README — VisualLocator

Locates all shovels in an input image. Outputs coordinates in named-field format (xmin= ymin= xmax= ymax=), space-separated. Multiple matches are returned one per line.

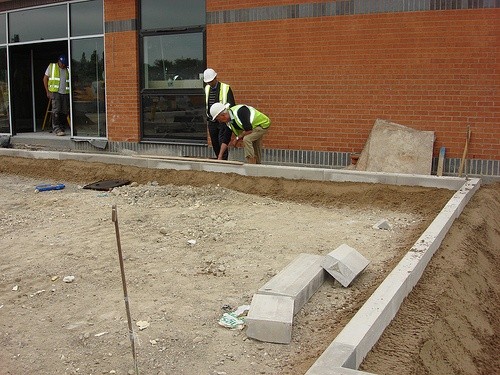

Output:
xmin=123 ymin=149 xmax=244 ymax=164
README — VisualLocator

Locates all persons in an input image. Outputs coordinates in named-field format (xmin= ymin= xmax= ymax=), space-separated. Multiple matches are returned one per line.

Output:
xmin=210 ymin=103 xmax=272 ymax=164
xmin=43 ymin=55 xmax=69 ymax=136
xmin=204 ymin=68 xmax=235 ymax=160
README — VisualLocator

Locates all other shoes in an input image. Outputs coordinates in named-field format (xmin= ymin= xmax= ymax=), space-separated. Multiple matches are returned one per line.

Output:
xmin=54 ymin=130 xmax=66 ymax=136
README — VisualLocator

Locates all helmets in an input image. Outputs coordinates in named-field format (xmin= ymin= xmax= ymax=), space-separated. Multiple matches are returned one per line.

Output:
xmin=204 ymin=69 xmax=217 ymax=82
xmin=58 ymin=55 xmax=68 ymax=65
xmin=209 ymin=103 xmax=230 ymax=120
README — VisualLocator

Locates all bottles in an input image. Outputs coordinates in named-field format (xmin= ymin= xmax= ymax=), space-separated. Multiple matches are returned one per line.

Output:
xmin=168 ymin=78 xmax=173 ymax=87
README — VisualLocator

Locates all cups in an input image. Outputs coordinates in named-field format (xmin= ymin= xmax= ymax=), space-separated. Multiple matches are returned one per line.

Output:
xmin=199 ymin=74 xmax=204 ymax=79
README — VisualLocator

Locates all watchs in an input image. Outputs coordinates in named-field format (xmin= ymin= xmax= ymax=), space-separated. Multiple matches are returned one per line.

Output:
xmin=236 ymin=137 xmax=239 ymax=140
